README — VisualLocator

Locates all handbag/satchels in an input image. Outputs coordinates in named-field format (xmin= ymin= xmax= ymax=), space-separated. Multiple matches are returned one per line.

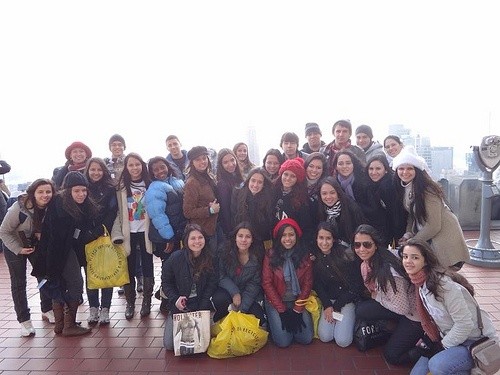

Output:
xmin=354 ymin=319 xmax=392 ymax=352
xmin=206 ymin=310 xmax=270 ymax=359
xmin=85 ymin=224 xmax=130 ymax=290
xmin=171 ymin=304 xmax=211 ymax=357
xmin=295 ymin=295 xmax=323 ymax=340
xmin=469 ymin=336 xmax=500 ymax=375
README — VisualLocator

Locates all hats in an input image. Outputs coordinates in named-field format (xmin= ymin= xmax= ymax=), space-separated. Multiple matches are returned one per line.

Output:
xmin=273 ymin=218 xmax=302 ymax=239
xmin=304 ymin=123 xmax=323 ymax=138
xmin=355 ymin=125 xmax=373 ymax=139
xmin=65 ymin=142 xmax=92 ymax=161
xmin=188 ymin=146 xmax=210 ymax=160
xmin=332 ymin=119 xmax=353 ymax=136
xmin=391 ymin=145 xmax=426 ymax=172
xmin=108 ymin=134 xmax=126 ymax=152
xmin=279 ymin=157 xmax=306 ymax=184
xmin=63 ymin=171 xmax=88 ymax=188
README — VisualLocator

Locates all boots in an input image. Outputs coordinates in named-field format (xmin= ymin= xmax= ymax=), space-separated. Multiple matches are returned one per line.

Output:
xmin=124 ymin=278 xmax=136 ymax=319
xmin=52 ymin=295 xmax=93 ymax=336
xmin=140 ymin=276 xmax=154 ymax=316
xmin=136 ymin=273 xmax=144 ymax=292
xmin=118 ymin=285 xmax=124 ymax=294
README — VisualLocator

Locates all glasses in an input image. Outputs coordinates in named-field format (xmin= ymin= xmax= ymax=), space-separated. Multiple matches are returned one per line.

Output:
xmin=352 ymin=241 xmax=375 ymax=249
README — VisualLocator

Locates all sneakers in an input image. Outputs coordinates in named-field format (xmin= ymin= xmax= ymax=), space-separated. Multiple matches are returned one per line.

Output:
xmin=41 ymin=309 xmax=56 ymax=324
xmin=88 ymin=303 xmax=110 ymax=325
xmin=19 ymin=319 xmax=36 ymax=337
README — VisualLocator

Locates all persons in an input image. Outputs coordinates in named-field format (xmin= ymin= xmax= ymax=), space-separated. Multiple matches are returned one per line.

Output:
xmin=0 ymin=120 xmax=471 ymax=365
xmin=399 ymin=238 xmax=497 ymax=375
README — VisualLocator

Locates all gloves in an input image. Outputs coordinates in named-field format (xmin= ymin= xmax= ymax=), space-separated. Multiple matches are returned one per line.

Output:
xmin=93 ymin=223 xmax=112 ymax=236
xmin=280 ymin=309 xmax=306 ymax=334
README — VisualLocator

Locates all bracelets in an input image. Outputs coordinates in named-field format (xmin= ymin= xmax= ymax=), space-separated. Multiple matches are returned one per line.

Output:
xmin=210 ymin=207 xmax=214 ymax=214
